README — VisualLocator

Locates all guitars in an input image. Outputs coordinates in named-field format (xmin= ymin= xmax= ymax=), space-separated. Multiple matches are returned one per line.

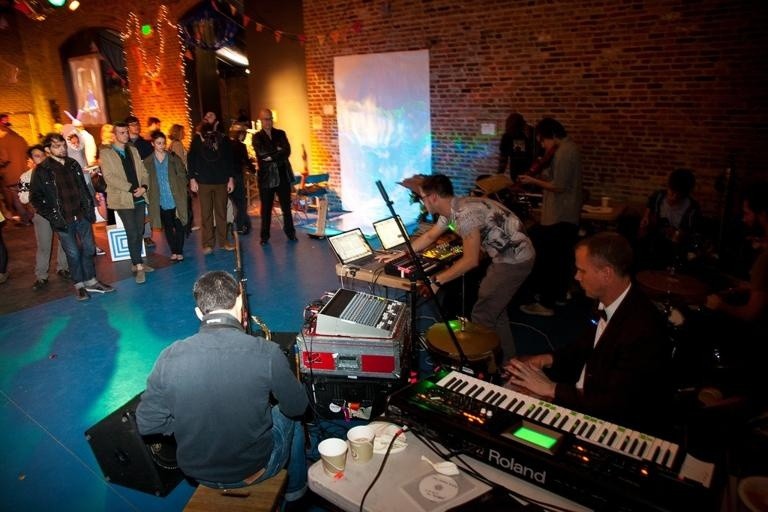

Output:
xmin=639 ymin=211 xmax=691 ymax=245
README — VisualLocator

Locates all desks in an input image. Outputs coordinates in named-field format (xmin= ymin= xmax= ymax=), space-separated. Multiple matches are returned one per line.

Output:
xmin=531 ymin=200 xmax=625 ymax=223
xmin=637 ymin=266 xmax=702 ymax=315
xmin=306 ymin=419 xmax=493 ymax=512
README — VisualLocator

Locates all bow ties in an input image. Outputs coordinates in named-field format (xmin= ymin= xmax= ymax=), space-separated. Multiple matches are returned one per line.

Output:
xmin=594 ymin=308 xmax=608 ymax=322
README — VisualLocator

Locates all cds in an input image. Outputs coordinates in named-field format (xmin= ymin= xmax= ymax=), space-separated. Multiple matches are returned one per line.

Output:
xmin=418 ymin=473 xmax=460 ymax=503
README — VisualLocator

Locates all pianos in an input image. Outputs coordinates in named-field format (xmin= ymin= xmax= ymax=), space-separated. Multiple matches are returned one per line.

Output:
xmin=385 ymin=368 xmax=727 ymax=512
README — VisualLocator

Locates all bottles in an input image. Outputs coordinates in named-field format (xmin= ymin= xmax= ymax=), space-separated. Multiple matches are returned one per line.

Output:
xmin=255 ymin=120 xmax=261 ymax=129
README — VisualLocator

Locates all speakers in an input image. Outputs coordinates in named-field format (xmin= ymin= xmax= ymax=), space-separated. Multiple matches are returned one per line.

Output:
xmin=85 ymin=391 xmax=187 ymax=499
xmin=252 ymin=331 xmax=299 ymax=406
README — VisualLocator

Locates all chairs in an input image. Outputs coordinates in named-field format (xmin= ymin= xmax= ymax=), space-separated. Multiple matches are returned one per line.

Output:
xmin=258 ymin=178 xmax=309 ymax=228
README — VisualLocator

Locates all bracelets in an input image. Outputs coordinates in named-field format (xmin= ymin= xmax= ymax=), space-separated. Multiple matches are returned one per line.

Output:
xmin=431 ymin=276 xmax=442 ymax=288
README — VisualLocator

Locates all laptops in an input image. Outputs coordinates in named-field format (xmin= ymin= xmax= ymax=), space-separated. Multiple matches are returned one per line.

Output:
xmin=373 ymin=215 xmax=436 ymax=257
xmin=326 ymin=227 xmax=404 ymax=274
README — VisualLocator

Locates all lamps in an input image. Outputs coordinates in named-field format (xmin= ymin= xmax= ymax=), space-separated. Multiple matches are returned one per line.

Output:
xmin=206 ymin=45 xmax=256 ymax=78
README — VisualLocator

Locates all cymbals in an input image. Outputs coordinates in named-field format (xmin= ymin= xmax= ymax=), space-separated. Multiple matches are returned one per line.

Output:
xmin=636 ymin=270 xmax=705 ymax=296
xmin=427 ymin=320 xmax=501 ymax=361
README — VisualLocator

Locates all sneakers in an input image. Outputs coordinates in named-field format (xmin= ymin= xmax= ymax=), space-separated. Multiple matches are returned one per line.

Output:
xmin=0 ymin=246 xmax=115 ymax=301
xmin=131 ymin=224 xmax=298 ymax=284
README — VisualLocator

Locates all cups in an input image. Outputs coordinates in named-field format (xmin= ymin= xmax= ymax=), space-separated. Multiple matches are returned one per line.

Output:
xmin=600 ymin=196 xmax=611 ymax=206
xmin=316 ymin=437 xmax=349 ymax=478
xmin=345 ymin=424 xmax=379 ymax=463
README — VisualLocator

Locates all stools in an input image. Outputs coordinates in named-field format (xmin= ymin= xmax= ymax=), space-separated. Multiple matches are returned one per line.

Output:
xmin=182 ymin=468 xmax=288 ymax=511
xmin=426 ymin=320 xmax=505 ymax=388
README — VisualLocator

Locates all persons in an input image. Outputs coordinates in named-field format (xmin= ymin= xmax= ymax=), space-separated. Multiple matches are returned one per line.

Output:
xmin=704 ymin=184 xmax=767 ymax=322
xmin=640 ymin=169 xmax=707 ymax=246
xmin=373 ymin=174 xmax=536 ymax=367
xmin=500 ymin=232 xmax=663 ymax=440
xmin=135 ymin=269 xmax=309 ymax=507
xmin=84 ymin=79 xmax=102 ymax=119
xmin=495 ymin=111 xmax=585 ymax=316
xmin=17 ymin=108 xmax=298 ymax=302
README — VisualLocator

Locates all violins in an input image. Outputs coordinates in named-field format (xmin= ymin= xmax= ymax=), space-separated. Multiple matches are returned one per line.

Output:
xmin=504 ymin=143 xmax=558 ymax=197
